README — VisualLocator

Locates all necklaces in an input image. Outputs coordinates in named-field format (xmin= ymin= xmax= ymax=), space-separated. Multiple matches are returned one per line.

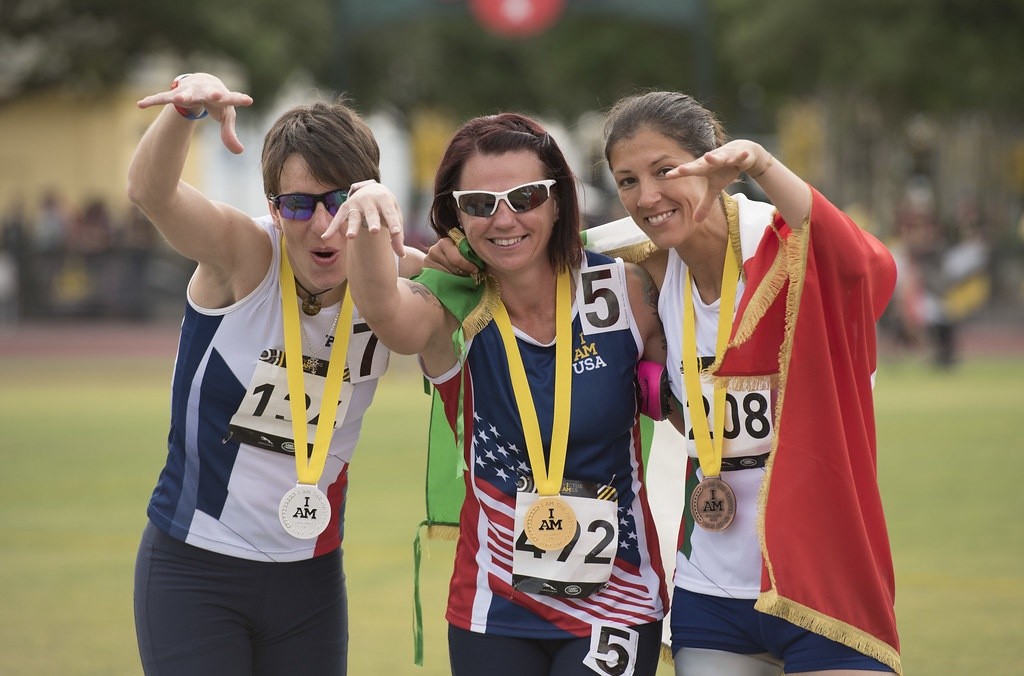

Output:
xmin=292 ymin=273 xmax=346 ymax=369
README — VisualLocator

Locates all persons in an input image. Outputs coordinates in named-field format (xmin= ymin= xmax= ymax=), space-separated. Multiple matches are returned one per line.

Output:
xmin=320 ymin=108 xmax=674 ymax=676
xmin=605 ymin=88 xmax=901 ymax=675
xmin=123 ymin=73 xmax=436 ymax=675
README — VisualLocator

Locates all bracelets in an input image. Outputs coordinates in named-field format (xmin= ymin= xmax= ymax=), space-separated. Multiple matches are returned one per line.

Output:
xmin=751 ymin=154 xmax=773 ymax=179
xmin=172 ymin=74 xmax=211 ymax=121
xmin=346 ymin=179 xmax=383 ymax=229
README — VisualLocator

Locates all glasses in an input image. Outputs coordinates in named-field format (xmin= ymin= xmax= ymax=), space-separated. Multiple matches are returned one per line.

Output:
xmin=266 ymin=187 xmax=354 ymax=219
xmin=450 ymin=178 xmax=557 ymax=218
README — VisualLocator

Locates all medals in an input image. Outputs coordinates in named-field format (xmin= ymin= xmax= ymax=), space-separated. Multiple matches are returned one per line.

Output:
xmin=523 ymin=495 xmax=578 ymax=551
xmin=689 ymin=474 xmax=737 ymax=533
xmin=278 ymin=479 xmax=332 ymax=541
